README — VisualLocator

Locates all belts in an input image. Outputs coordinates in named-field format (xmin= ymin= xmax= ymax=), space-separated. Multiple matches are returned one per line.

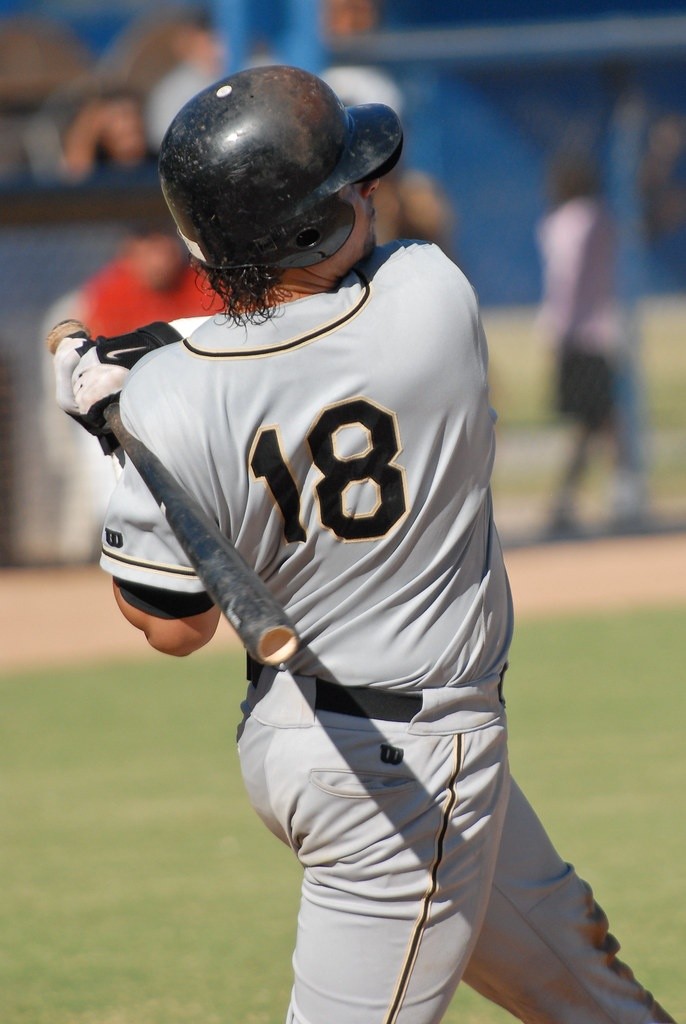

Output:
xmin=246 ymin=654 xmax=423 ymax=724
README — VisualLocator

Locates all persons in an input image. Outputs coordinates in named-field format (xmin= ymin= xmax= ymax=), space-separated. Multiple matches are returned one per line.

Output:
xmin=51 ymin=66 xmax=680 ymax=1024
xmin=0 ymin=0 xmax=686 ymax=536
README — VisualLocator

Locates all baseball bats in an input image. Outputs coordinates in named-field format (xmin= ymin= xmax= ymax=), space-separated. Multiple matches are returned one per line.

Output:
xmin=45 ymin=319 xmax=301 ymax=670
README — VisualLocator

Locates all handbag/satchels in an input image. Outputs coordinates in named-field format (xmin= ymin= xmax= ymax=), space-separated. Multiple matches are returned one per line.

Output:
xmin=558 ymin=346 xmax=616 ymax=421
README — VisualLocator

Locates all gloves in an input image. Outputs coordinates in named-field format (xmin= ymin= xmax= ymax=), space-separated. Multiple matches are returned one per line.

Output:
xmin=52 ymin=332 xmax=120 ymax=454
xmin=72 ymin=321 xmax=184 ymax=434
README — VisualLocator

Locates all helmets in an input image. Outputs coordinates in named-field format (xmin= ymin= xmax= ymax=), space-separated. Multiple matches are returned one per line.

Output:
xmin=156 ymin=65 xmax=405 ymax=270
